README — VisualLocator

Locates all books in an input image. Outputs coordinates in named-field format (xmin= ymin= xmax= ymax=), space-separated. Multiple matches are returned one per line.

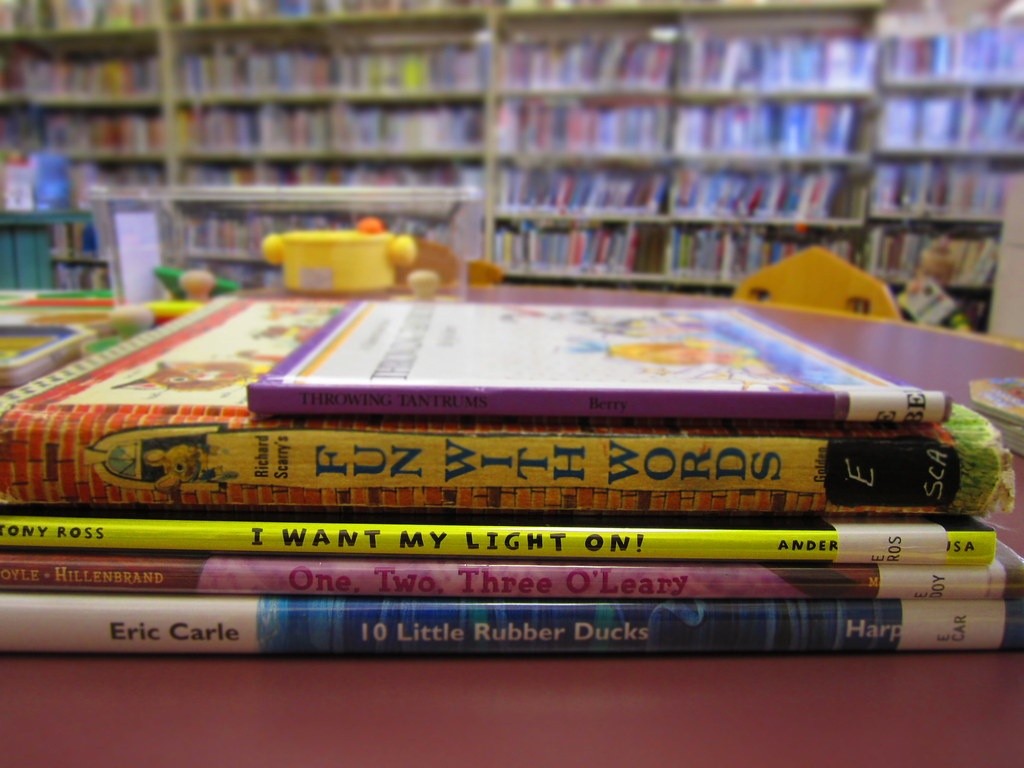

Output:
xmin=0 ymin=0 xmax=1024 ymax=333
xmin=0 ymin=295 xmax=1024 ymax=652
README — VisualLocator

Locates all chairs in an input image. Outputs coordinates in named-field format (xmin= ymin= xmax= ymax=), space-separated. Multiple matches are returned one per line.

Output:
xmin=730 ymin=246 xmax=905 ymax=323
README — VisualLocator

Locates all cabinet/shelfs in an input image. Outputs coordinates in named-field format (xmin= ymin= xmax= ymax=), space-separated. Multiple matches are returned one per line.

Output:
xmin=0 ymin=0 xmax=1024 ymax=336
